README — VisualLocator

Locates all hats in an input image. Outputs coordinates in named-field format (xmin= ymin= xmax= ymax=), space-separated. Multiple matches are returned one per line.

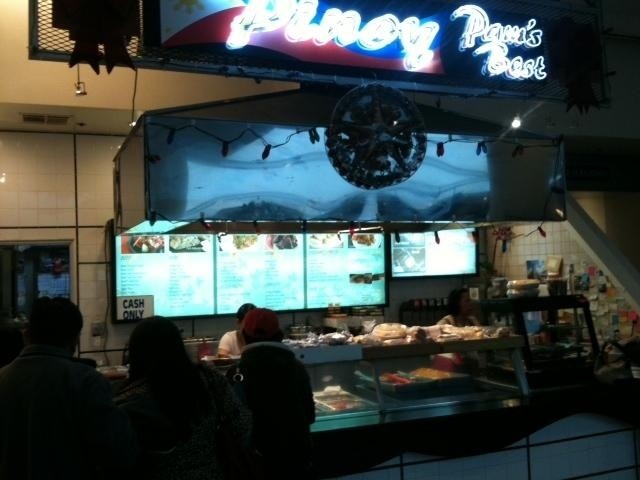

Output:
xmin=243 ymin=308 xmax=278 ymax=337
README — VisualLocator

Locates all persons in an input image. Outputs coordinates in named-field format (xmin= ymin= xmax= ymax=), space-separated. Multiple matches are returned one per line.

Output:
xmin=436 ymin=288 xmax=479 ymax=326
